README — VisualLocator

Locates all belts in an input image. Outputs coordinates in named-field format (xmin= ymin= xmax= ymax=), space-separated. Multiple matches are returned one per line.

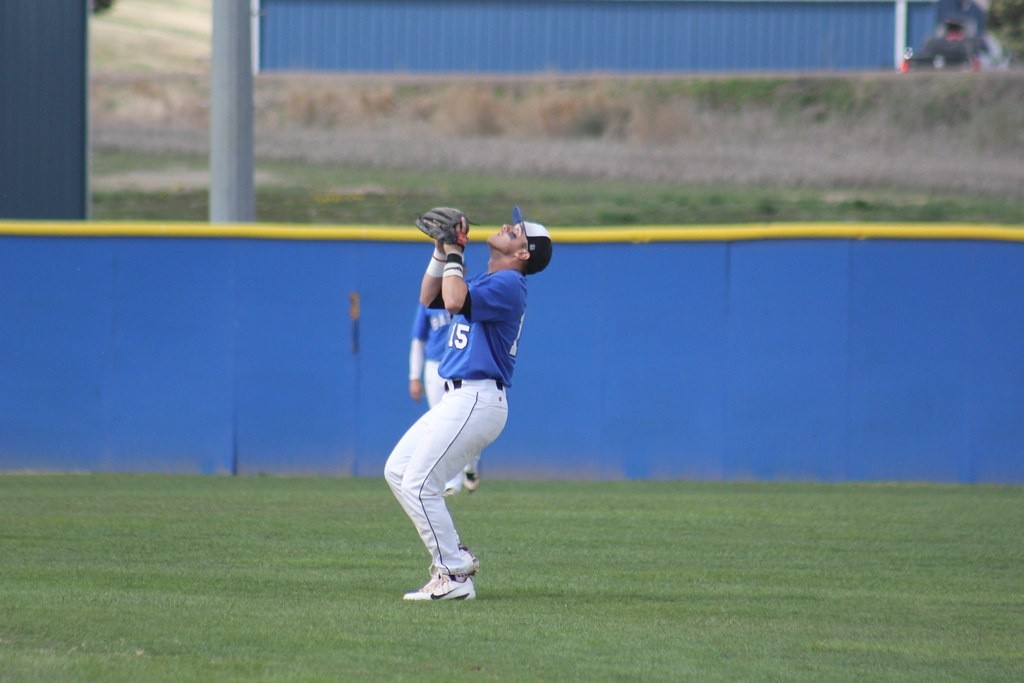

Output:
xmin=444 ymin=379 xmax=503 ymax=391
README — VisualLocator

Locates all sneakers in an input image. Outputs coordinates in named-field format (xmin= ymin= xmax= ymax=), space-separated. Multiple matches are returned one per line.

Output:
xmin=458 ymin=545 xmax=480 ymax=575
xmin=403 ymin=575 xmax=476 ymax=600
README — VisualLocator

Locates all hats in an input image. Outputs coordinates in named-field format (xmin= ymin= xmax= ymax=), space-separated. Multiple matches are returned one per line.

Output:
xmin=512 ymin=205 xmax=552 ymax=276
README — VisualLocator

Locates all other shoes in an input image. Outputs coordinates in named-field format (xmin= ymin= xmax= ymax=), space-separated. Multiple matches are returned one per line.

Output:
xmin=465 ymin=472 xmax=480 ymax=491
xmin=444 ymin=488 xmax=460 ymax=495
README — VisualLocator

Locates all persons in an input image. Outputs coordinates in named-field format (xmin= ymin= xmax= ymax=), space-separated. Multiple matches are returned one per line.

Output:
xmin=409 ymin=305 xmax=480 ymax=496
xmin=384 ymin=206 xmax=552 ymax=600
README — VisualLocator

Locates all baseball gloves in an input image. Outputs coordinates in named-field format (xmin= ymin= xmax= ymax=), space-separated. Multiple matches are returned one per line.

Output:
xmin=413 ymin=206 xmax=479 ymax=245
